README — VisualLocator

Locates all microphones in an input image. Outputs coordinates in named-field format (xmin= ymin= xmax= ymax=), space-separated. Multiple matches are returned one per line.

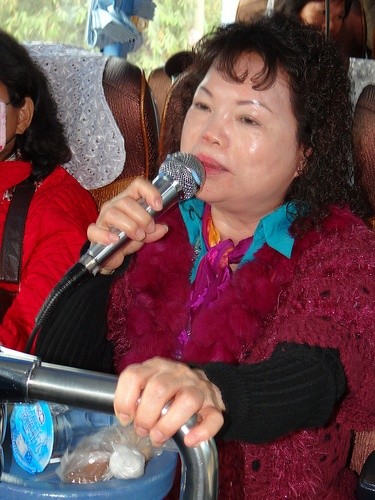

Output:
xmin=75 ymin=152 xmax=206 ymax=278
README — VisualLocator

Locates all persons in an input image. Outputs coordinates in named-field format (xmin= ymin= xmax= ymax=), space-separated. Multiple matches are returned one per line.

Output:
xmin=165 ymin=51 xmax=198 ymax=88
xmin=1 ymin=26 xmax=99 ymax=354
xmin=35 ymin=12 xmax=373 ymax=499
xmin=276 ymin=1 xmax=350 ymax=37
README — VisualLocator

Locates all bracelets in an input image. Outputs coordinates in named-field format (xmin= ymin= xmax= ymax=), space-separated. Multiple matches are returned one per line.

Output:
xmin=99 ymin=268 xmax=115 ymax=275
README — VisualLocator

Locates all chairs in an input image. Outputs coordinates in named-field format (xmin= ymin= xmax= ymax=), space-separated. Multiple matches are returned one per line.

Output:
xmin=19 ymin=40 xmax=159 ymax=218
xmin=160 ymin=61 xmax=375 ymax=228
